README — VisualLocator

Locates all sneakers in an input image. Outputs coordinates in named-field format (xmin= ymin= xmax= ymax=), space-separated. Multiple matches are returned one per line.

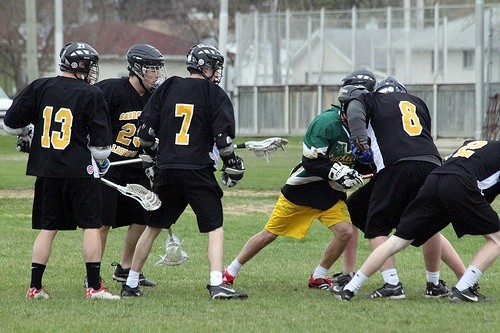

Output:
xmin=307 ymin=274 xmax=333 ymax=290
xmin=448 ymin=282 xmax=492 ymax=303
xmin=366 ymin=281 xmax=406 ymax=300
xmin=111 ymin=261 xmax=156 ymax=287
xmin=206 ymin=282 xmax=248 ymax=300
xmin=26 ymin=287 xmax=50 ymax=300
xmin=84 ymin=275 xmax=107 ymax=288
xmin=424 ymin=280 xmax=450 ymax=298
xmin=329 ymin=272 xmax=355 ymax=286
xmin=86 ymin=282 xmax=120 ymax=300
xmin=120 ymin=281 xmax=144 ymax=298
xmin=329 ymin=283 xmax=355 ymax=302
xmin=222 ymin=267 xmax=235 ymax=289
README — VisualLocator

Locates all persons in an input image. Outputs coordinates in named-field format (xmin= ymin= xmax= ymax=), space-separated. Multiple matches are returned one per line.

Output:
xmin=3 ymin=41 xmax=122 ymax=299
xmin=121 ymin=43 xmax=248 ymax=299
xmin=84 ymin=44 xmax=168 ymax=289
xmin=222 ymin=69 xmax=486 ymax=300
xmin=331 ymin=140 xmax=500 ymax=303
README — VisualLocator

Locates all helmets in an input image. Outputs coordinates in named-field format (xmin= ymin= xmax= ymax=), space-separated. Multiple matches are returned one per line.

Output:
xmin=186 ymin=42 xmax=225 ymax=73
xmin=337 ymin=84 xmax=369 ymax=103
xmin=373 ymin=76 xmax=407 ymax=94
xmin=126 ymin=45 xmax=165 ymax=80
xmin=59 ymin=42 xmax=99 ymax=73
xmin=341 ymin=69 xmax=377 ymax=90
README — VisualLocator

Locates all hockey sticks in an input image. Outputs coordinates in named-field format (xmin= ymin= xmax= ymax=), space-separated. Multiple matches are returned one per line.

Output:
xmin=99 ymin=176 xmax=163 ymax=213
xmin=103 ymin=134 xmax=289 ymax=166
xmin=140 ymin=153 xmax=187 ymax=268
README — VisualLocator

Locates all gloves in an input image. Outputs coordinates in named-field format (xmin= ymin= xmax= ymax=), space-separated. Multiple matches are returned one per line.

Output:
xmin=16 ymin=127 xmax=33 ymax=153
xmin=221 ymin=156 xmax=245 ymax=188
xmin=138 ymin=153 xmax=156 ymax=183
xmin=351 ymin=142 xmax=374 ymax=165
xmin=327 ymin=161 xmax=364 ymax=189
xmin=94 ymin=159 xmax=110 ymax=177
